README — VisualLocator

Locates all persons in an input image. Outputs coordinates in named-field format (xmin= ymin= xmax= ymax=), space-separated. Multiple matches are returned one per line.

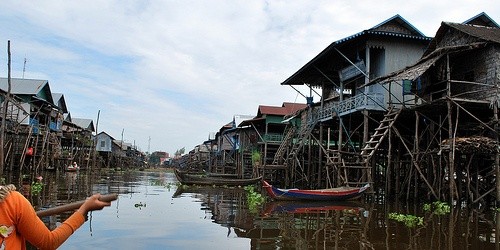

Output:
xmin=0 ymin=184 xmax=111 ymax=250
xmin=73 ymin=161 xmax=78 ymax=168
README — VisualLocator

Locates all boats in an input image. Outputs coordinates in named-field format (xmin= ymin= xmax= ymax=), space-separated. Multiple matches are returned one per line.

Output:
xmin=66 ymin=162 xmax=80 ymax=172
xmin=262 ymin=180 xmax=371 ymax=201
xmin=174 ymin=168 xmax=263 ymax=185
